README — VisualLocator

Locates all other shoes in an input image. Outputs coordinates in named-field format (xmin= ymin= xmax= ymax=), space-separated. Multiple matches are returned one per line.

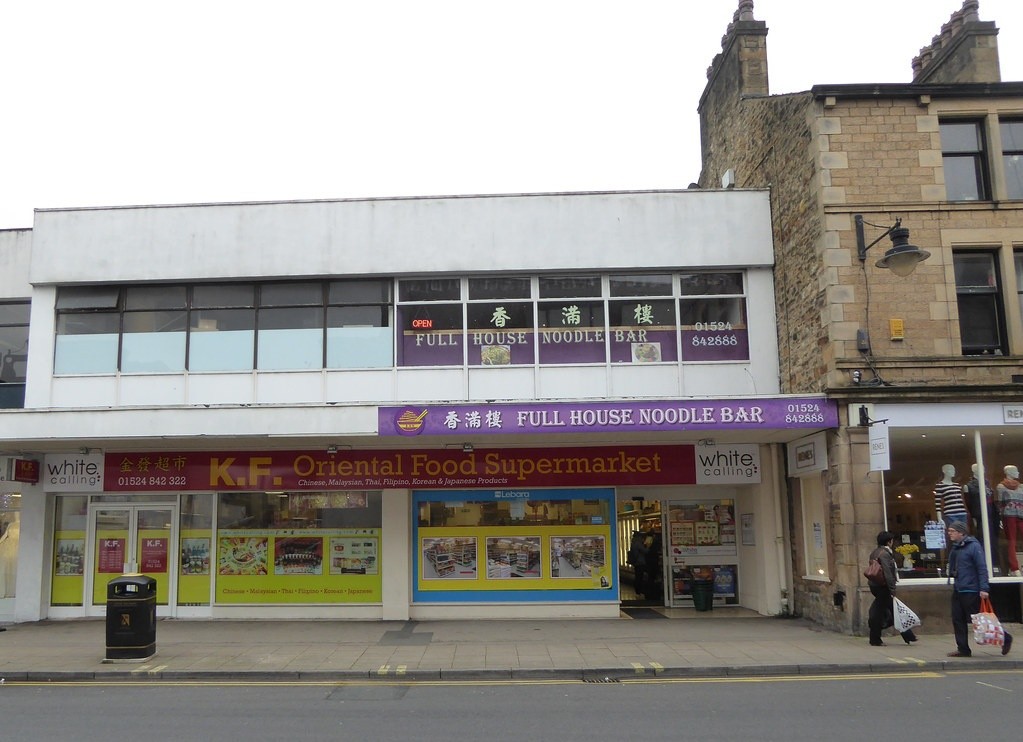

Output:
xmin=946 ymin=650 xmax=972 ymax=657
xmin=1001 ymin=632 xmax=1013 ymax=654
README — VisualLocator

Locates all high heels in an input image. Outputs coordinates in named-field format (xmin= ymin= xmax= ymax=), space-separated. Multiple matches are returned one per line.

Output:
xmin=869 ymin=640 xmax=888 ymax=645
xmin=904 ymin=637 xmax=918 ymax=644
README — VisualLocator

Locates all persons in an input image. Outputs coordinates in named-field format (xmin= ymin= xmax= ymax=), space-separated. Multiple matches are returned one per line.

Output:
xmin=933 ymin=463 xmax=968 ymax=578
xmin=995 ymin=464 xmax=1022 ymax=576
xmin=947 ymin=521 xmax=1013 ymax=658
xmin=963 ymin=463 xmax=1003 ymax=576
xmin=867 ymin=531 xmax=919 ymax=646
xmin=630 ymin=521 xmax=657 ymax=599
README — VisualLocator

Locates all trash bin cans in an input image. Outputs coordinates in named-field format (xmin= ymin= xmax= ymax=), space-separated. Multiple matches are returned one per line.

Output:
xmin=692 ymin=580 xmax=714 ymax=610
xmin=105 ymin=572 xmax=159 ymax=662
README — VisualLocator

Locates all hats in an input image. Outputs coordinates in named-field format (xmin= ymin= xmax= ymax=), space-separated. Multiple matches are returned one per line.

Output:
xmin=950 ymin=521 xmax=970 ymax=535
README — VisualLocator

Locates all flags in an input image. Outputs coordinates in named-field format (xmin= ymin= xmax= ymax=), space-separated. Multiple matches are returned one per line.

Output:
xmin=867 ymin=424 xmax=891 ymax=472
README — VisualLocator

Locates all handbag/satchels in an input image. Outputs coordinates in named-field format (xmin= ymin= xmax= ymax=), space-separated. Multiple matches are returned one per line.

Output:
xmin=863 ymin=558 xmax=886 ymax=585
xmin=887 ymin=592 xmax=922 ymax=632
xmin=971 ymin=596 xmax=1003 ymax=645
xmin=867 ymin=599 xmax=894 ymax=630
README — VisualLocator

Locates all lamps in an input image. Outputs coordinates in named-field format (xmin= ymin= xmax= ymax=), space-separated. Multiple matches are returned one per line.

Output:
xmin=860 ymin=217 xmax=931 ymax=278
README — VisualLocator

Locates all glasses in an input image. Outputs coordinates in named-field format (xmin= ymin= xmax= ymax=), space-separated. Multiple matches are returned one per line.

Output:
xmin=947 ymin=529 xmax=959 ymax=533
